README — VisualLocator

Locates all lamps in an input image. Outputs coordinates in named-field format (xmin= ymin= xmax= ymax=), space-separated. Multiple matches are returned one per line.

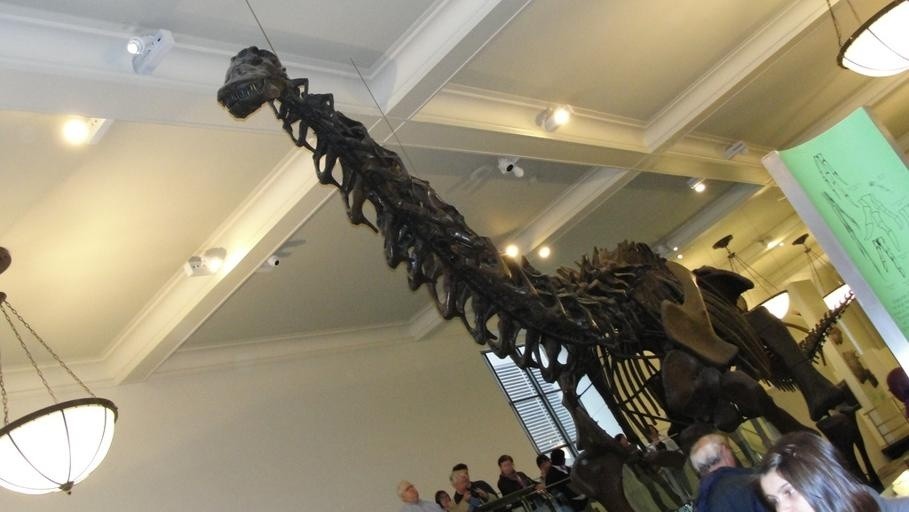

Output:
xmin=126 ymin=29 xmax=176 ymax=76
xmin=827 ymin=0 xmax=909 ymax=79
xmin=0 ymin=244 xmax=118 ymax=494
xmin=712 ymin=233 xmax=855 ymax=321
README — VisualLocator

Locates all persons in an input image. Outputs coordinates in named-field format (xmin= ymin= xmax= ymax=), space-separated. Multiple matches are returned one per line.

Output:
xmin=642 ymin=424 xmax=695 ymax=511
xmin=690 ymin=434 xmax=775 ymax=512
xmin=886 ymin=367 xmax=909 ymax=426
xmin=393 ymin=448 xmax=594 ymax=512
xmin=756 ymin=431 xmax=909 ymax=512
xmin=615 ymin=433 xmax=690 ymax=511
